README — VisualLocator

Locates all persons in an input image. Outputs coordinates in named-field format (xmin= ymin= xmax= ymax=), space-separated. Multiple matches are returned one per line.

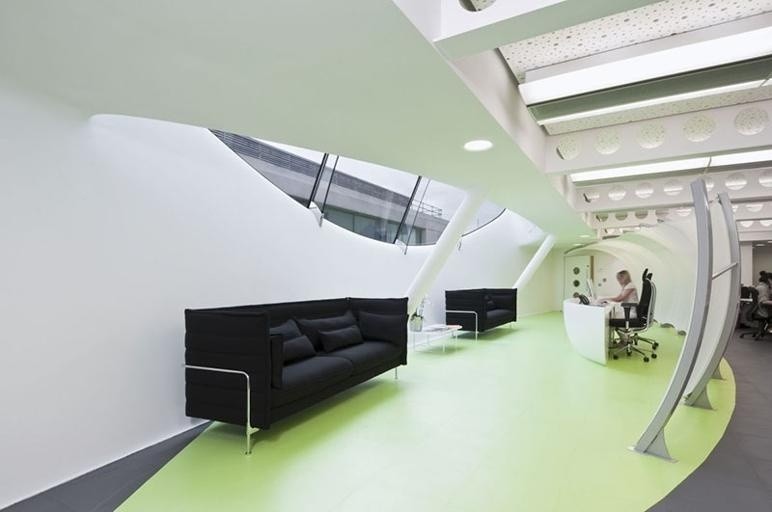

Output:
xmin=597 ymin=270 xmax=639 ymax=347
xmin=753 ymin=271 xmax=772 ymax=334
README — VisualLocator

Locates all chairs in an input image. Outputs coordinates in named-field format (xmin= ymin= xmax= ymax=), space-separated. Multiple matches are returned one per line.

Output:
xmin=444 ymin=287 xmax=517 ymax=336
xmin=739 ymin=286 xmax=772 ymax=342
xmin=608 ymin=268 xmax=659 ymax=364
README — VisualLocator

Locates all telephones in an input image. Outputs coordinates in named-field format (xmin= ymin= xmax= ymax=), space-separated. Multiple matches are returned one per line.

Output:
xmin=580 ymin=295 xmax=589 ymax=304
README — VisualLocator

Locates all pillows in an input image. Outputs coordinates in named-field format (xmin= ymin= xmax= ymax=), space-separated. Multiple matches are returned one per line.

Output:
xmin=266 ymin=310 xmax=408 ymax=390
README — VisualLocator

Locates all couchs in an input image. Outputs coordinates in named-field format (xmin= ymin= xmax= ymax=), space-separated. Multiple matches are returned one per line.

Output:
xmin=183 ymin=297 xmax=410 ymax=456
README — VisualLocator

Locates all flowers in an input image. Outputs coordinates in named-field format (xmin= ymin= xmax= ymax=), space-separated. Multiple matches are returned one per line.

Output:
xmin=409 ymin=312 xmax=424 ymax=332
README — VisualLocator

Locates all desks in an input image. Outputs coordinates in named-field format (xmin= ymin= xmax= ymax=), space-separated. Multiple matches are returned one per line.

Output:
xmin=407 ymin=324 xmax=461 ymax=354
